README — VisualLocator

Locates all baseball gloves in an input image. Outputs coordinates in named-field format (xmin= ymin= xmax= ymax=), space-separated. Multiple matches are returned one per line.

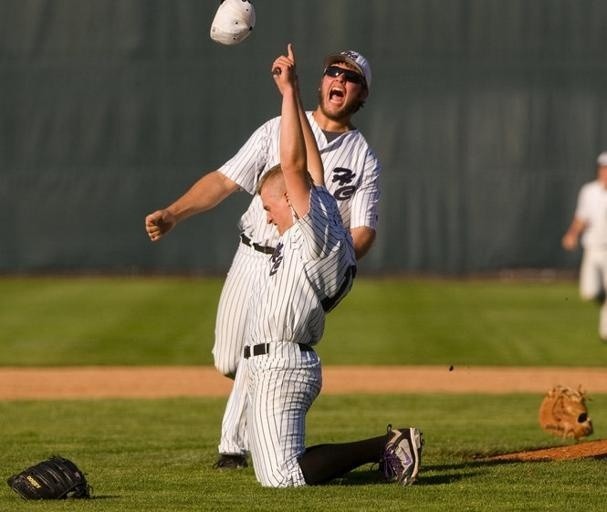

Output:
xmin=7 ymin=455 xmax=94 ymax=499
xmin=539 ymin=384 xmax=592 ymax=438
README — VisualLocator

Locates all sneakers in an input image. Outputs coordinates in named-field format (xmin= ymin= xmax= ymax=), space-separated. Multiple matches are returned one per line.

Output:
xmin=377 ymin=423 xmax=426 ymax=489
xmin=212 ymin=453 xmax=249 ymax=471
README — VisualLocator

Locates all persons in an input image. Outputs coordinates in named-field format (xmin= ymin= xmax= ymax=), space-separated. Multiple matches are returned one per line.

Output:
xmin=560 ymin=151 xmax=606 ymax=343
xmin=237 ymin=43 xmax=423 ymax=490
xmin=142 ymin=54 xmax=379 ymax=467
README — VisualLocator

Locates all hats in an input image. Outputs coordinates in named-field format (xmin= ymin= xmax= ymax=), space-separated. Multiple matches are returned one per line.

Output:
xmin=597 ymin=150 xmax=607 ymax=167
xmin=207 ymin=0 xmax=259 ymax=47
xmin=322 ymin=48 xmax=374 ymax=92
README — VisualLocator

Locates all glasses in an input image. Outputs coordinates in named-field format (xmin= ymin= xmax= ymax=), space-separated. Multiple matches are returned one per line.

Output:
xmin=323 ymin=65 xmax=366 ymax=88
